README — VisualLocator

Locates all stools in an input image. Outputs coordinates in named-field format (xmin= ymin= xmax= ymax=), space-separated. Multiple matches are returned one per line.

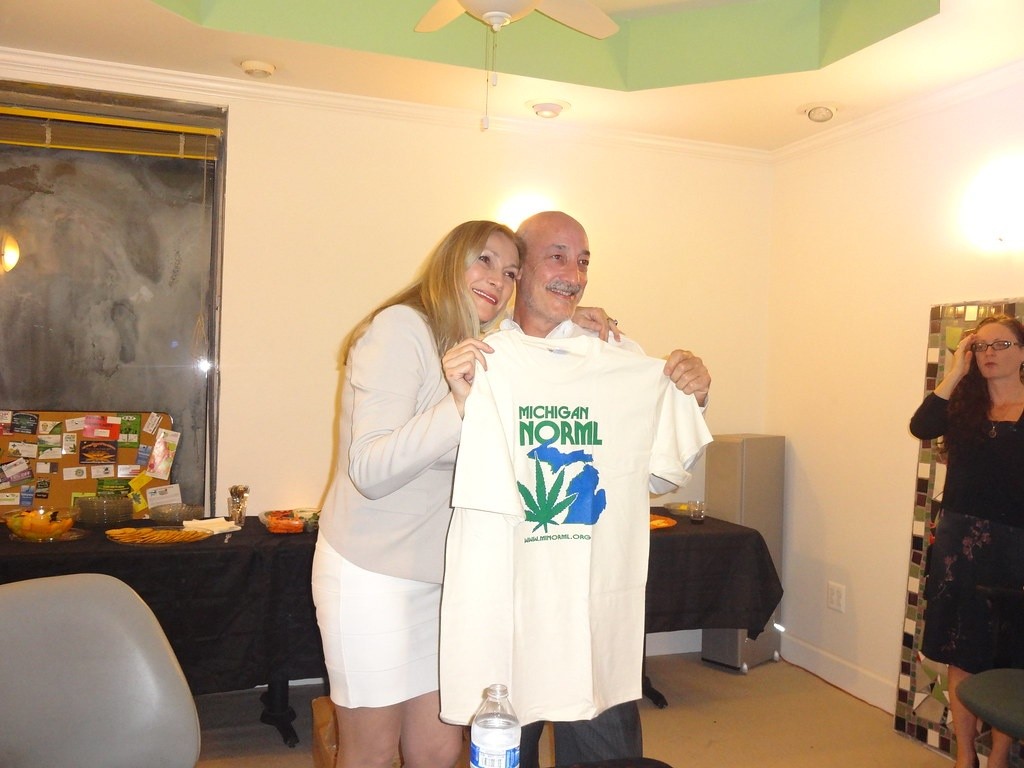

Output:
xmin=955 ymin=668 xmax=1024 ymax=739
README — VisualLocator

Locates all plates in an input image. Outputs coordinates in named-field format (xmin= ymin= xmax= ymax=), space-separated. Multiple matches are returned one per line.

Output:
xmin=105 ymin=525 xmax=214 ymax=547
xmin=76 ymin=495 xmax=132 ymax=523
xmin=150 ymin=504 xmax=205 ymax=522
xmin=664 ymin=503 xmax=703 ymax=516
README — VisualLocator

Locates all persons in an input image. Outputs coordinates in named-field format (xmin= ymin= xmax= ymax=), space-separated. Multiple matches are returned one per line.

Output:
xmin=910 ymin=315 xmax=1024 ymax=768
xmin=311 ymin=220 xmax=621 ymax=768
xmin=467 ymin=211 xmax=711 ymax=768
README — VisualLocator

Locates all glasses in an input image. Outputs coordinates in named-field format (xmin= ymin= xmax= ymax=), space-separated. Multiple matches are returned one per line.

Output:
xmin=971 ymin=340 xmax=1021 ymax=353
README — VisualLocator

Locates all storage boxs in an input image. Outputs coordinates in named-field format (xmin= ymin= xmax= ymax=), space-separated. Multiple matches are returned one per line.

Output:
xmin=311 ymin=696 xmax=403 ymax=768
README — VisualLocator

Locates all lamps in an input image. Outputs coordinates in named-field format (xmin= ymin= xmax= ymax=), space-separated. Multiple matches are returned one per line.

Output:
xmin=0 ymin=223 xmax=20 ymax=275
xmin=235 ymin=55 xmax=284 ymax=78
xmin=526 ymin=98 xmax=571 ymax=119
xmin=796 ymin=100 xmax=846 ymax=124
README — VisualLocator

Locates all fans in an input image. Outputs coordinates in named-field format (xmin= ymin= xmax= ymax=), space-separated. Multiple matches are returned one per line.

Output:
xmin=414 ymin=0 xmax=619 ymax=131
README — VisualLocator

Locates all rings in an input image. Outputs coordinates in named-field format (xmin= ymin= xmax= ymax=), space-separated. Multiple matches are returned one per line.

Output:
xmin=606 ymin=317 xmax=618 ymax=326
xmin=957 ymin=344 xmax=960 ymax=349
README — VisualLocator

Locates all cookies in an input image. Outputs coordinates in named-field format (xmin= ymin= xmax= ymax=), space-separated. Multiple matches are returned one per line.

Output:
xmin=105 ymin=528 xmax=210 ymax=543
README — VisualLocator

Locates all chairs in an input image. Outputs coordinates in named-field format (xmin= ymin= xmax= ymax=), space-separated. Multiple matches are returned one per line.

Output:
xmin=0 ymin=574 xmax=200 ymax=768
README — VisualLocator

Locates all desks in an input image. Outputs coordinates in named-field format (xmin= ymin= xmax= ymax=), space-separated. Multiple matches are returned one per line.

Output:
xmin=0 ymin=504 xmax=783 ymax=750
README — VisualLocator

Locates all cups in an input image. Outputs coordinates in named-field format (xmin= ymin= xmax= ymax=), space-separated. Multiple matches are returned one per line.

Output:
xmin=688 ymin=500 xmax=705 ymax=525
xmin=227 ymin=497 xmax=247 ymax=526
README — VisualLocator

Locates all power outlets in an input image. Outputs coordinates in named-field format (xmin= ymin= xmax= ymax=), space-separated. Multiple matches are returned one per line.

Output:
xmin=828 ymin=581 xmax=846 ymax=613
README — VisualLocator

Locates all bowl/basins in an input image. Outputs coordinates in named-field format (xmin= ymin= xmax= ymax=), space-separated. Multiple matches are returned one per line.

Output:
xmin=2 ymin=506 xmax=82 ymax=538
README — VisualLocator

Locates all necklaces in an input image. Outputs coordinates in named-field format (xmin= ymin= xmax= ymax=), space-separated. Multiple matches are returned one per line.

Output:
xmin=989 ymin=402 xmax=1016 ymax=438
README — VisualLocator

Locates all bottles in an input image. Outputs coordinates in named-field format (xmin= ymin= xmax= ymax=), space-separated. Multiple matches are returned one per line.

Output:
xmin=470 ymin=684 xmax=520 ymax=768
